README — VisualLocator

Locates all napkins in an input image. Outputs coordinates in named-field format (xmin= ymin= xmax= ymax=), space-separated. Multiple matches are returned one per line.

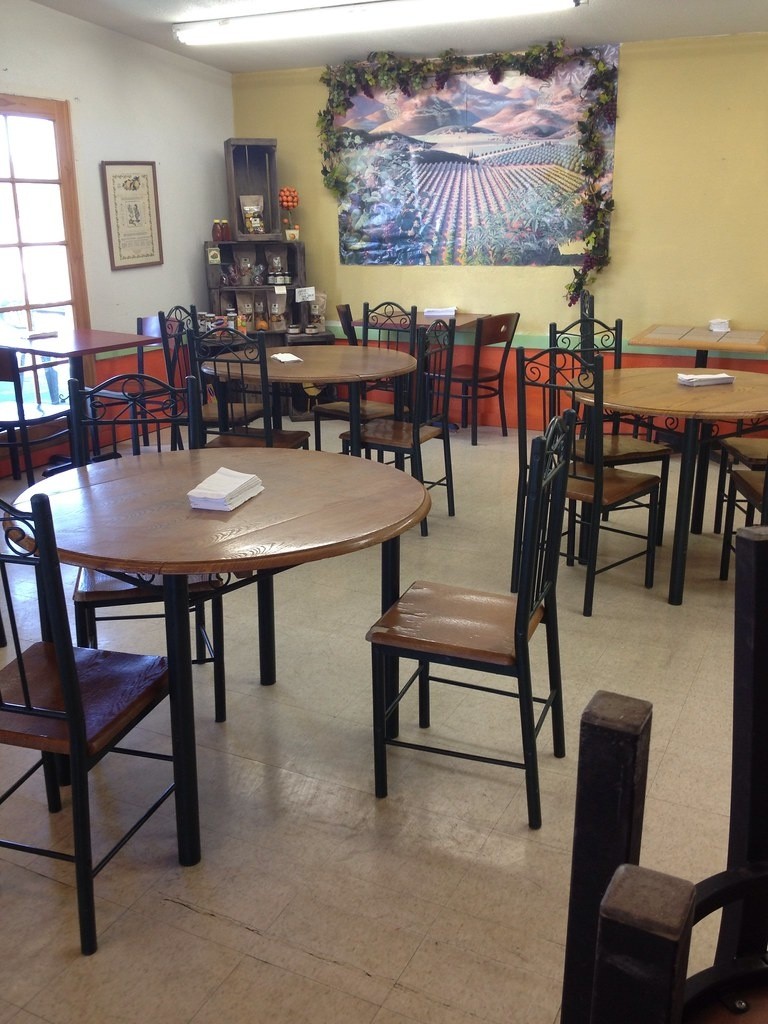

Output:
xmin=271 ymin=353 xmax=303 ymax=363
xmin=709 ymin=319 xmax=730 ymax=332
xmin=22 ymin=331 xmax=58 ymax=338
xmin=424 ymin=306 xmax=457 ymax=315
xmin=676 ymin=373 xmax=735 ymax=387
xmin=186 ymin=466 xmax=265 ymax=511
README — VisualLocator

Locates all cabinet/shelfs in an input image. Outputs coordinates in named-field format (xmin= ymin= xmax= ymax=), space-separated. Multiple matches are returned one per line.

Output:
xmin=194 ymin=138 xmax=340 ymax=422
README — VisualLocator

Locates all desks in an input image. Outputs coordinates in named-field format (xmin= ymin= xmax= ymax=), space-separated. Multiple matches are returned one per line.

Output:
xmin=628 ymin=323 xmax=768 ymax=368
xmin=201 ymin=345 xmax=417 ymax=457
xmin=351 ymin=311 xmax=491 ymax=440
xmin=567 ymin=368 xmax=768 ymax=606
xmin=16 ymin=446 xmax=430 ymax=866
xmin=0 ymin=329 xmax=162 ymax=478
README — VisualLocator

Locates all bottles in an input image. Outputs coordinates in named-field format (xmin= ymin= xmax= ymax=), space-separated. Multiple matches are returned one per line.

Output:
xmin=205 ymin=314 xmax=216 ymax=338
xmin=267 ymin=272 xmax=275 ymax=285
xmin=197 ymin=312 xmax=207 ymax=332
xmin=221 ymin=220 xmax=231 ymax=241
xmin=275 ymin=272 xmax=284 ymax=285
xmin=283 ymin=272 xmax=292 ymax=285
xmin=227 ymin=313 xmax=237 ymax=337
xmin=226 ymin=308 xmax=235 ymax=316
xmin=212 ymin=220 xmax=223 ymax=241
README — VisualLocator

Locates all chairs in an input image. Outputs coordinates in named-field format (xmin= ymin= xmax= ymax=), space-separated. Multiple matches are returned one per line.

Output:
xmin=0 ymin=301 xmax=768 ymax=1024
